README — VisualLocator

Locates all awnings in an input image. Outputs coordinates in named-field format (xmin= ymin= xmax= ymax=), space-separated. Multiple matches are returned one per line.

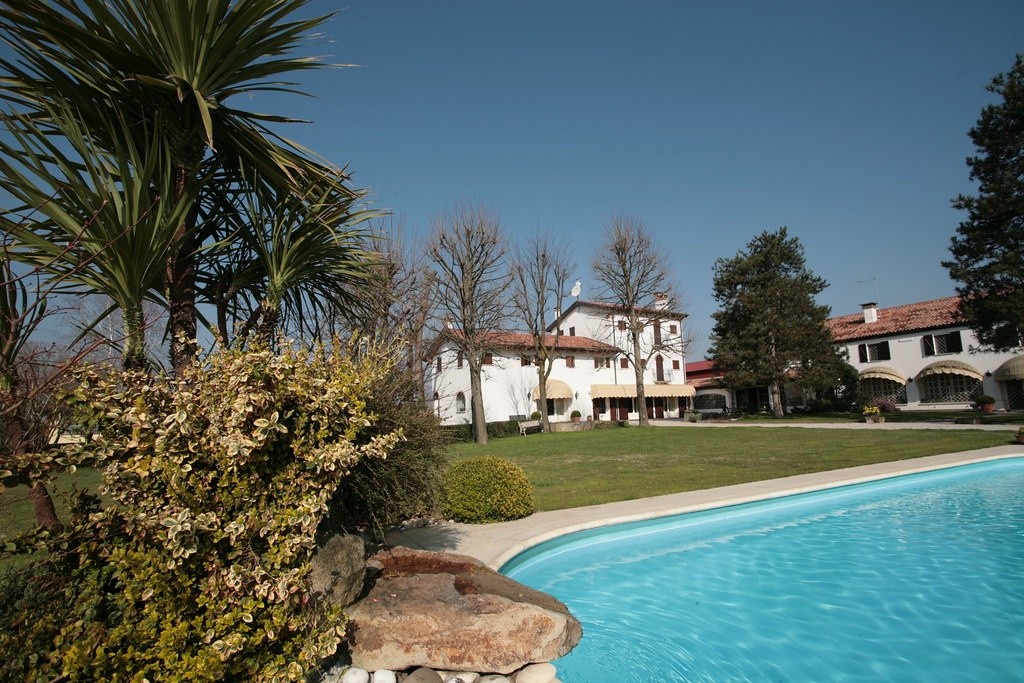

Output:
xmin=995 ymin=355 xmax=1024 ymax=382
xmin=916 ymin=360 xmax=984 ymax=382
xmin=859 ymin=367 xmax=906 ymax=385
xmin=590 ymin=384 xmax=696 ymax=400
xmin=532 ymin=379 xmax=573 ymax=401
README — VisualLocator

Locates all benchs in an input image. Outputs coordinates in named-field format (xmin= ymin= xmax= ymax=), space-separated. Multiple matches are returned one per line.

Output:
xmin=517 ymin=420 xmax=544 ymax=437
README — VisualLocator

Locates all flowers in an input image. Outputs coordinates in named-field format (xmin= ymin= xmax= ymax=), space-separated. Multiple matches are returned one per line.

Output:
xmin=862 ymin=406 xmax=880 ymax=416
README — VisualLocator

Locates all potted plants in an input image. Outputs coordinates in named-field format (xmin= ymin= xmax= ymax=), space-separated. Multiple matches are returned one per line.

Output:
xmin=975 ymin=395 xmax=995 ymax=413
xmin=571 ymin=411 xmax=581 ymax=422
xmin=1014 ymin=426 xmax=1024 ymax=443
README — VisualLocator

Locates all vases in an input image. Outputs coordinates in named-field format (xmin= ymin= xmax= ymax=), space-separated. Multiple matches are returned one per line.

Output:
xmin=864 ymin=415 xmax=884 ymax=423
